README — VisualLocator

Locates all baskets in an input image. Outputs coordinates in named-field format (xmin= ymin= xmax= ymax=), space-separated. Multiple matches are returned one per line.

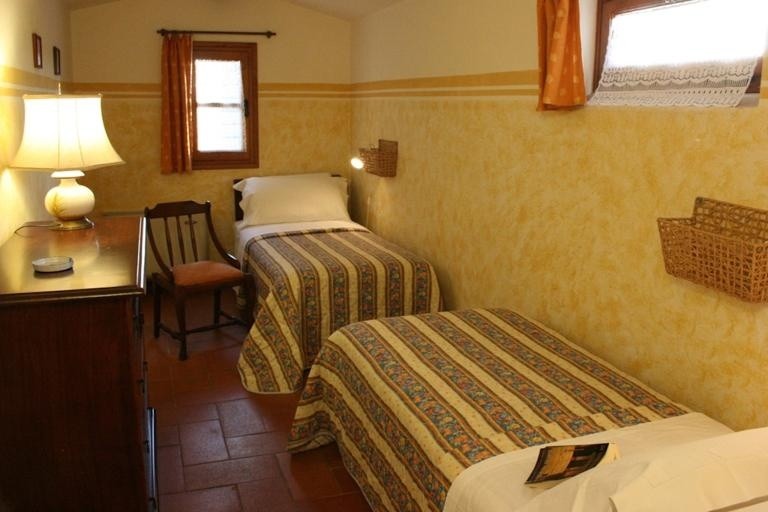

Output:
xmin=656 ymin=196 xmax=768 ymax=303
xmin=359 ymin=140 xmax=398 ymax=179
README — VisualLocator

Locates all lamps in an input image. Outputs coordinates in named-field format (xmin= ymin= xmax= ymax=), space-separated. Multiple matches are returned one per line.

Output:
xmin=9 ymin=90 xmax=126 ymax=234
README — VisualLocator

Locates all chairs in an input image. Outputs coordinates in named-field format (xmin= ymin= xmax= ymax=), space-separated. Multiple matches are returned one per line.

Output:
xmin=140 ymin=197 xmax=242 ymax=362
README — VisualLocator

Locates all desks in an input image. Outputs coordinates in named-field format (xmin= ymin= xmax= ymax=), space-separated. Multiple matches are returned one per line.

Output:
xmin=2 ymin=211 xmax=157 ymax=510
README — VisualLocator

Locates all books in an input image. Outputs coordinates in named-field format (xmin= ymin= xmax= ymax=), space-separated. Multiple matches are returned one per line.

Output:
xmin=522 ymin=442 xmax=620 ymax=492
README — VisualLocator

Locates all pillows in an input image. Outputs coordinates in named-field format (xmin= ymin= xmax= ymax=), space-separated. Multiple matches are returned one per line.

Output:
xmin=233 ymin=174 xmax=358 ymax=219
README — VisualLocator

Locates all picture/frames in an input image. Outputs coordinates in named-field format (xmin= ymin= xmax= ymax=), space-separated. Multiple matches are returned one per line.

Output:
xmin=53 ymin=45 xmax=72 ymax=76
xmin=31 ymin=35 xmax=46 ymax=73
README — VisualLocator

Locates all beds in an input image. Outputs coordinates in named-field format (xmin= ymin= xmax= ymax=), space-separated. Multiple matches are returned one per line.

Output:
xmin=284 ymin=306 xmax=767 ymax=510
xmin=232 ymin=170 xmax=439 ymax=395
